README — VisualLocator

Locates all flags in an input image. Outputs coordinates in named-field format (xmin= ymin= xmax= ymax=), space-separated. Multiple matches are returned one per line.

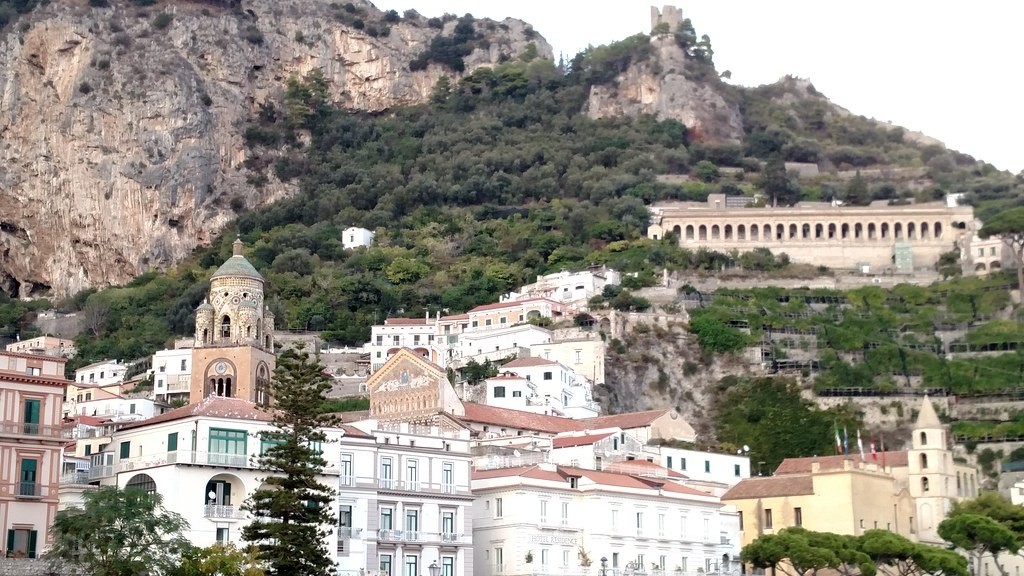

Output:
xmin=834 ymin=421 xmax=843 ymax=453
xmin=844 ymin=429 xmax=877 ymax=461
